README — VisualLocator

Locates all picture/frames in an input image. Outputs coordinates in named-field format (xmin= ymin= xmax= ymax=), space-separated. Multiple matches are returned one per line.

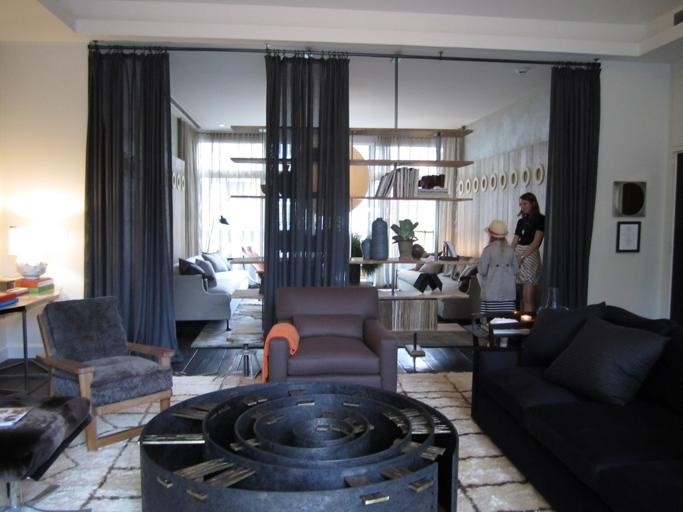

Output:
xmin=617 ymin=222 xmax=641 ymax=252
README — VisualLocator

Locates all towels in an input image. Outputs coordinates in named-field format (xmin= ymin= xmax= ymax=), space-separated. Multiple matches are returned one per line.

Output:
xmin=260 ymin=322 xmax=300 ymax=384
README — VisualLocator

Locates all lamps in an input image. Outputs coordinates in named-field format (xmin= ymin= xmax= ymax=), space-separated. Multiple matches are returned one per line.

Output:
xmin=9 ymin=226 xmax=49 ymax=281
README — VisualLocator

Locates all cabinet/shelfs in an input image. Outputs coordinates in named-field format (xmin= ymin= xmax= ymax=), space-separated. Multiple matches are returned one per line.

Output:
xmin=228 ymin=126 xmax=475 ymax=298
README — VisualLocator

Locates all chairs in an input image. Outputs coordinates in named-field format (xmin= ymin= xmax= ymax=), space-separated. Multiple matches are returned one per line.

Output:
xmin=35 ymin=295 xmax=177 ymax=451
xmin=0 ymin=390 xmax=92 ymax=512
xmin=270 ymin=286 xmax=398 ymax=394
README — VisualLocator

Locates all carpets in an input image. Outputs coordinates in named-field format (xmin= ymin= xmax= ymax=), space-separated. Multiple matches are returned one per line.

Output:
xmin=189 ymin=289 xmax=473 ymax=348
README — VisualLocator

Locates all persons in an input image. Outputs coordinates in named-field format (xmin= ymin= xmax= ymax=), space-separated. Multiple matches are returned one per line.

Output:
xmin=512 ymin=193 xmax=545 ymax=313
xmin=475 ymin=219 xmax=518 ymax=348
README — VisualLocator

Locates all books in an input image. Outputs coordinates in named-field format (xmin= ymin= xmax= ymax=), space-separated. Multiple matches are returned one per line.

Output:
xmin=374 ymin=168 xmax=448 ymax=198
xmin=0 ymin=279 xmax=54 ymax=308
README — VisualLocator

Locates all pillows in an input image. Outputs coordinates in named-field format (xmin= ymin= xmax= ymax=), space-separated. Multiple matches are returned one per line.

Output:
xmin=292 ymin=314 xmax=364 ymax=340
xmin=543 ymin=316 xmax=671 ymax=407
xmin=178 ymin=250 xmax=232 ymax=293
xmin=412 ymin=259 xmax=477 ymax=293
xmin=518 ymin=303 xmax=609 ymax=366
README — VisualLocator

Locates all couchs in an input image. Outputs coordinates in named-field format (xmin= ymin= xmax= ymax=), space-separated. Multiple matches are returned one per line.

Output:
xmin=397 ymin=255 xmax=482 ymax=322
xmin=471 ymin=302 xmax=682 ymax=512
xmin=172 ymin=250 xmax=247 ymax=332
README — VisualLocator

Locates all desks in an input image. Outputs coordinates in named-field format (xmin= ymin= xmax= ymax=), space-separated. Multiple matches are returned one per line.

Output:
xmin=0 ymin=288 xmax=61 ymax=396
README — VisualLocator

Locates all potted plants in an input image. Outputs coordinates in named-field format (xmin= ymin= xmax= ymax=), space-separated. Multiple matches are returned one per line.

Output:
xmin=391 ymin=220 xmax=419 ymax=257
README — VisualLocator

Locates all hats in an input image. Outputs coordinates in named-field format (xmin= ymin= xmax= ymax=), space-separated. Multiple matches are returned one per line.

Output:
xmin=485 ymin=221 xmax=508 ymax=237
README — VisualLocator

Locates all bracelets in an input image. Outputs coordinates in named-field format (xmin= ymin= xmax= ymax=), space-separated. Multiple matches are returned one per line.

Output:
xmin=519 ymin=255 xmax=525 ymax=260
